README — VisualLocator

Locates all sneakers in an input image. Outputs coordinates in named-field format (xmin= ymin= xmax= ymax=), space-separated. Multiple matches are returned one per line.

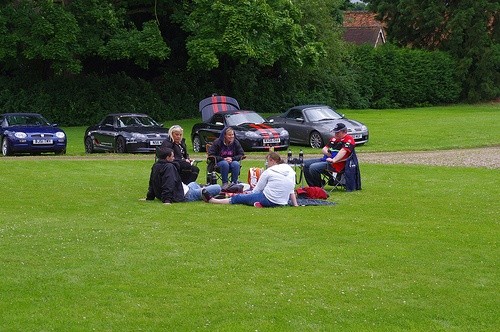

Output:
xmin=202 ymin=189 xmax=212 ymax=203
xmin=254 ymin=201 xmax=262 ymax=207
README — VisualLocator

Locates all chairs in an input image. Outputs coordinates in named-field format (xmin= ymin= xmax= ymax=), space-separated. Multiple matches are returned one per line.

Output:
xmin=321 ymin=148 xmax=356 ymax=193
xmin=205 ymin=143 xmax=247 ymax=184
xmin=155 ymin=144 xmax=203 ymax=166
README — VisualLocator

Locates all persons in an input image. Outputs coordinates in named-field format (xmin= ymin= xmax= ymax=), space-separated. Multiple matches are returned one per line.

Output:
xmin=160 ymin=125 xmax=200 ymax=184
xmin=303 ymin=123 xmax=355 ymax=189
xmin=207 ymin=127 xmax=245 ymax=191
xmin=139 ymin=148 xmax=222 ymax=205
xmin=202 ymin=153 xmax=299 ymax=207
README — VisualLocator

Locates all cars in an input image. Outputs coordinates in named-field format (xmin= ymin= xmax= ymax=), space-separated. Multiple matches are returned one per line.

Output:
xmin=191 ymin=96 xmax=291 ymax=152
xmin=83 ymin=112 xmax=170 ymax=153
xmin=266 ymin=105 xmax=369 ymax=149
xmin=0 ymin=113 xmax=67 ymax=156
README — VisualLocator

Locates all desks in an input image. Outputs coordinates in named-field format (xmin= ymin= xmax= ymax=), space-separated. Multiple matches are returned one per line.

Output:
xmin=265 ymin=162 xmax=305 ymax=187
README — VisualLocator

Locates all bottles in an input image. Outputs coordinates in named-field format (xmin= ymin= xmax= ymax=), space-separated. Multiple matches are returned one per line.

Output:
xmin=287 ymin=149 xmax=292 ymax=164
xmin=299 ymin=149 xmax=303 ymax=164
xmin=212 ymin=175 xmax=217 ymax=185
xmin=207 ymin=173 xmax=212 ymax=185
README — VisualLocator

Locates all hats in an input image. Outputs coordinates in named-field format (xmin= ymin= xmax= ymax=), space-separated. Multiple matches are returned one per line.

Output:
xmin=331 ymin=123 xmax=346 ymax=133
